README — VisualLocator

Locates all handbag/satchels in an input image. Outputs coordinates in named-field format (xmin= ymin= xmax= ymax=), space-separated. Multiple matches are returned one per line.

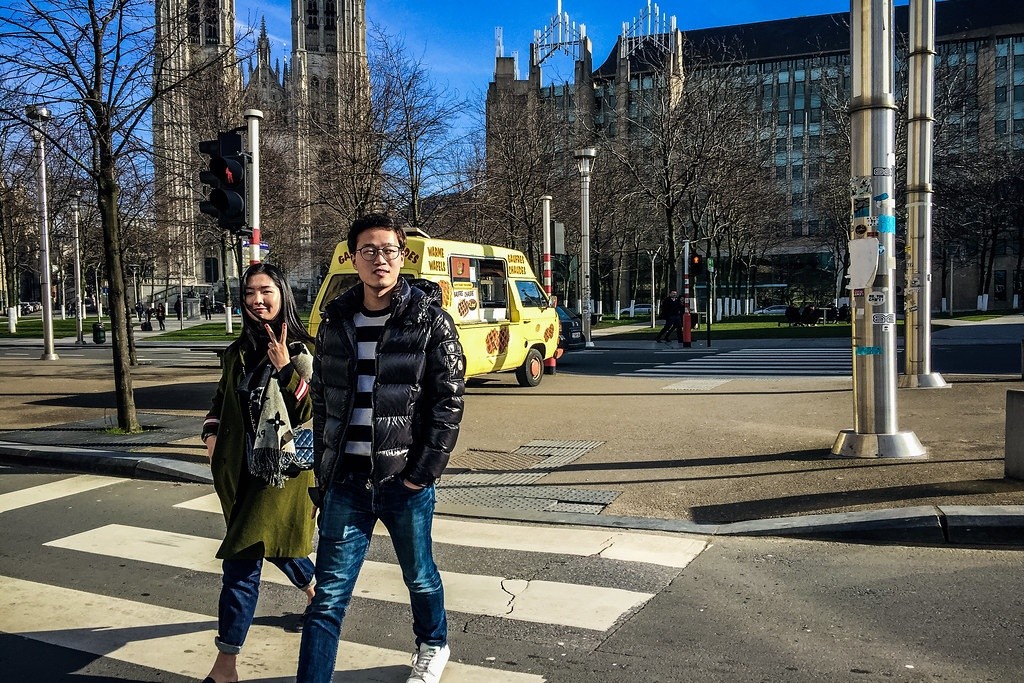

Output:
xmin=282 ymin=427 xmax=314 ymax=472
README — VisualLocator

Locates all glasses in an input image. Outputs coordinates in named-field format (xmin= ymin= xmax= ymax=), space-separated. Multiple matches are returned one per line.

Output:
xmin=355 ymin=244 xmax=402 ymax=261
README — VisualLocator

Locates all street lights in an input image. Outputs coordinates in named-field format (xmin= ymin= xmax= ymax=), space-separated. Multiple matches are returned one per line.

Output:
xmin=128 ymin=263 xmax=140 ymax=320
xmin=67 ymin=188 xmax=87 ymax=346
xmin=573 ymin=146 xmax=598 ymax=349
xmin=945 ymin=243 xmax=961 ymax=317
xmin=25 ymin=104 xmax=59 ymax=360
xmin=176 ymin=262 xmax=184 ymax=329
xmin=88 ymin=260 xmax=103 ymax=323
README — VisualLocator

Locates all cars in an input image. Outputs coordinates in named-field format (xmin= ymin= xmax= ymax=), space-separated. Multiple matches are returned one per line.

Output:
xmin=20 ymin=301 xmax=42 ymax=316
xmin=620 ymin=304 xmax=651 ymax=315
xmin=754 ymin=305 xmax=789 ymax=314
xmin=201 ymin=300 xmax=226 ymax=314
xmin=84 ymin=296 xmax=95 ymax=312
xmin=554 ymin=302 xmax=586 ymax=354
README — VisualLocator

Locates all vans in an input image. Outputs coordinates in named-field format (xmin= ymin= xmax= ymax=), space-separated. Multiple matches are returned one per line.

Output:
xmin=307 ymin=227 xmax=561 ymax=388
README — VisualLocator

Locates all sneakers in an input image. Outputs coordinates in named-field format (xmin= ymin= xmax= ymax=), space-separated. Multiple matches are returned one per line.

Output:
xmin=405 ymin=642 xmax=450 ymax=683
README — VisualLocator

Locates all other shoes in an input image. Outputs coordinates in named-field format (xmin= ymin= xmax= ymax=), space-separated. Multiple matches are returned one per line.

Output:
xmin=664 ymin=338 xmax=671 ymax=342
xmin=656 ymin=338 xmax=661 ymax=343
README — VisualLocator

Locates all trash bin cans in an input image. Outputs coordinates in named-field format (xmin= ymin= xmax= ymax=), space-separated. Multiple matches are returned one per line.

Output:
xmin=91 ymin=322 xmax=106 ymax=344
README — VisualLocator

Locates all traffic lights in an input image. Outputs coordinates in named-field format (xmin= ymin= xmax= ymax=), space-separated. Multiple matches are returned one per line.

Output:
xmin=199 ymin=134 xmax=244 ymax=225
xmin=209 ymin=154 xmax=247 ymax=230
xmin=689 ymin=254 xmax=703 ymax=268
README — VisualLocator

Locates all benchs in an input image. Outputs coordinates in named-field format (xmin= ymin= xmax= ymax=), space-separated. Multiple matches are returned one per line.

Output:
xmin=777 ymin=319 xmax=813 ymax=327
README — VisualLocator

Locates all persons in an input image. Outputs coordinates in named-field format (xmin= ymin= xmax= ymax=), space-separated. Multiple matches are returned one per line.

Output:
xmin=296 ymin=213 xmax=465 ymax=683
xmin=656 ymin=290 xmax=685 ymax=343
xmin=174 ymin=296 xmax=181 ymax=320
xmin=802 ymin=305 xmax=818 ymax=327
xmin=21 ymin=303 xmax=39 ymax=315
xmin=67 ymin=301 xmax=73 ymax=318
xmin=202 ymin=295 xmax=213 ymax=320
xmin=156 ymin=303 xmax=165 ymax=331
xmin=200 ymin=263 xmax=319 ymax=683
xmin=825 ymin=302 xmax=839 ymax=324
xmin=785 ymin=304 xmax=801 ymax=326
xmin=839 ymin=303 xmax=851 ymax=323
xmin=135 ymin=301 xmax=143 ymax=319
xmin=145 ymin=306 xmax=155 ymax=322
